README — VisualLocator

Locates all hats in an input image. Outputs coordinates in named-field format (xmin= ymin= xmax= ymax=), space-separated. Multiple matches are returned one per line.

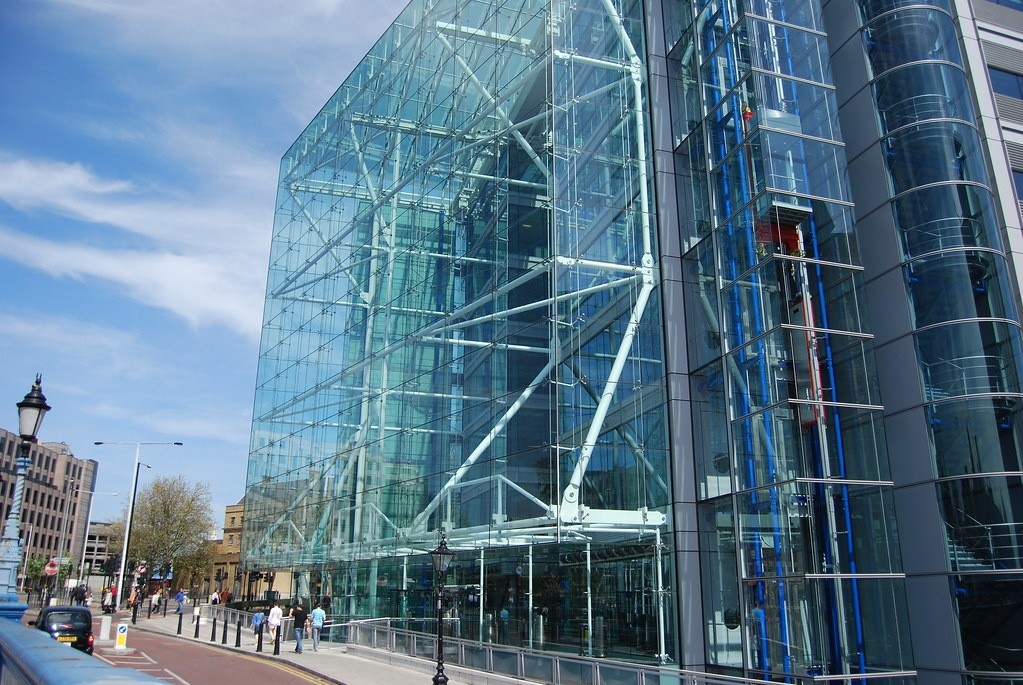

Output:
xmin=295 ymin=604 xmax=304 ymax=608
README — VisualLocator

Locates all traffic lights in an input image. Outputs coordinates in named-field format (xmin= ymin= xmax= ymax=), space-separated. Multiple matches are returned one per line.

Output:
xmin=263 ymin=574 xmax=268 ymax=582
xmin=237 ymin=571 xmax=243 ymax=578
xmin=216 ymin=569 xmax=221 ymax=578
xmin=248 ymin=572 xmax=255 ymax=582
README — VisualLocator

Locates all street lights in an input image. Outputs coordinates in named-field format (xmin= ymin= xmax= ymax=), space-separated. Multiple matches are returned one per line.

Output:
xmin=119 ymin=462 xmax=152 ymax=608
xmin=94 ymin=440 xmax=184 ymax=610
xmin=0 ymin=372 xmax=52 ymax=621
xmin=429 ymin=533 xmax=454 ymax=685
xmin=70 ymin=487 xmax=118 ymax=588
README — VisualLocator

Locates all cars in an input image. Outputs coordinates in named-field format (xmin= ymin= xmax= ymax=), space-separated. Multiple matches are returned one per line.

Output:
xmin=27 ymin=605 xmax=95 ymax=657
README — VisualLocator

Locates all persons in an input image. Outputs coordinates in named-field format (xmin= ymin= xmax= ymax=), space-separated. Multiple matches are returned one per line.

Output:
xmin=211 ymin=587 xmax=229 ymax=615
xmin=309 ymin=603 xmax=327 ymax=652
xmin=267 ymin=602 xmax=283 ymax=644
xmin=288 ymin=604 xmax=312 ymax=654
xmin=68 ymin=614 xmax=81 ymax=624
xmin=500 ymin=605 xmax=510 ymax=645
xmin=101 ymin=582 xmax=117 ymax=614
xmin=250 ymin=608 xmax=265 ymax=645
xmin=447 ymin=603 xmax=467 ymax=645
xmin=126 ymin=586 xmax=169 ymax=619
xmin=174 ymin=587 xmax=186 ymax=615
xmin=72 ymin=583 xmax=94 ymax=607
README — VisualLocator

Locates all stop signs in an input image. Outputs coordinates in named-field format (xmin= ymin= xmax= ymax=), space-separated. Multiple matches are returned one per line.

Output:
xmin=45 ymin=562 xmax=59 ymax=576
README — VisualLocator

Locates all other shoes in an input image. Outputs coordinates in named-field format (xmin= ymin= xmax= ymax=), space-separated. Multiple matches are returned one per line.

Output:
xmin=271 ymin=639 xmax=275 ymax=645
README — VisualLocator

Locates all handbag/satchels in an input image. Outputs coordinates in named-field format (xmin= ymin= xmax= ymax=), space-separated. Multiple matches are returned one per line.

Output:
xmin=158 ymin=597 xmax=161 ymax=605
